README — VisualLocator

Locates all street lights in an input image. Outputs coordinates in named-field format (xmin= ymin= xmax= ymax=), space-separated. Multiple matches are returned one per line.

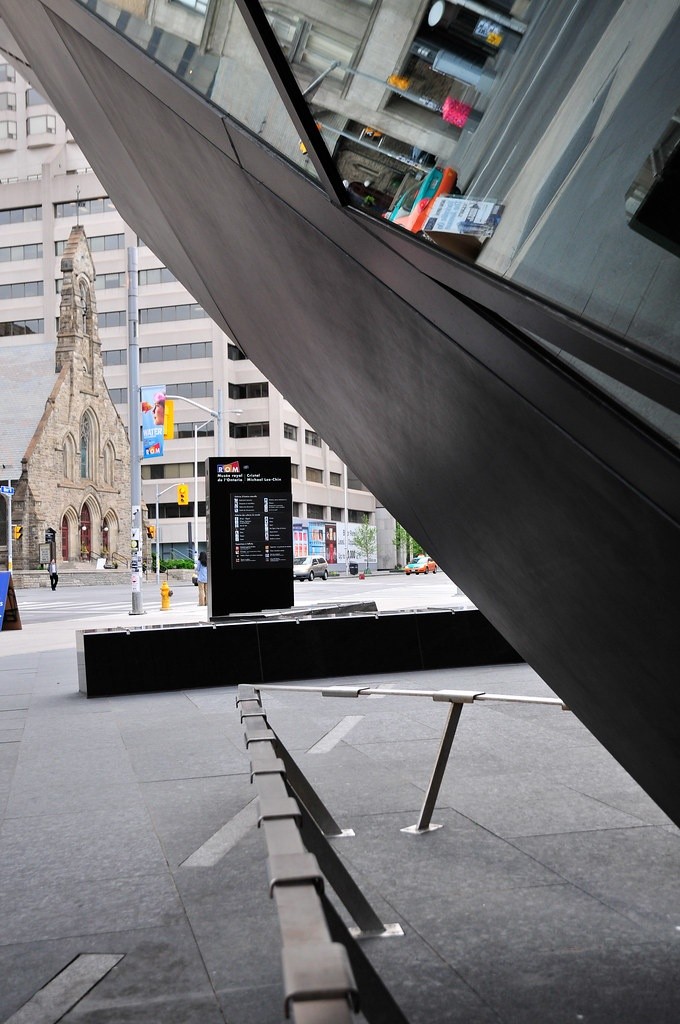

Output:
xmin=194 ymin=408 xmax=245 ymax=569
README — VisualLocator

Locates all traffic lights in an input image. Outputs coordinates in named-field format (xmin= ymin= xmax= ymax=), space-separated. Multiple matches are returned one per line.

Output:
xmin=177 ymin=482 xmax=189 ymax=506
xmin=14 ymin=525 xmax=22 ymax=540
xmin=146 ymin=525 xmax=155 ymax=538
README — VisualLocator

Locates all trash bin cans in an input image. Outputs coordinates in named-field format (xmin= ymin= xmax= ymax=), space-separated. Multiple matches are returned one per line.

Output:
xmin=349 ymin=563 xmax=358 ymax=575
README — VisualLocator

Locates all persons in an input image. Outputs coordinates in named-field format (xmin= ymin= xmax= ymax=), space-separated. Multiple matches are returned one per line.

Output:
xmin=195 ymin=552 xmax=208 ymax=606
xmin=47 ymin=559 xmax=59 ymax=591
xmin=328 ymin=542 xmax=334 ymax=563
xmin=151 ymin=392 xmax=165 ymax=426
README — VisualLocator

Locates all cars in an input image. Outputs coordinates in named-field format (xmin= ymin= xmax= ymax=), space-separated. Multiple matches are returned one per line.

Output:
xmin=405 ymin=555 xmax=438 ymax=575
xmin=292 ymin=555 xmax=329 ymax=582
xmin=192 ymin=575 xmax=198 ymax=586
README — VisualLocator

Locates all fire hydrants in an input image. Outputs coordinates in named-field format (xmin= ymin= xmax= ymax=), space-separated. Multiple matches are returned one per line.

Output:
xmin=159 ymin=581 xmax=174 ymax=610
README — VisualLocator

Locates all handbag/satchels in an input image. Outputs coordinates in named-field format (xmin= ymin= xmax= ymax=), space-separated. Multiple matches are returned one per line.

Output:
xmin=192 ymin=574 xmax=198 ymax=586
